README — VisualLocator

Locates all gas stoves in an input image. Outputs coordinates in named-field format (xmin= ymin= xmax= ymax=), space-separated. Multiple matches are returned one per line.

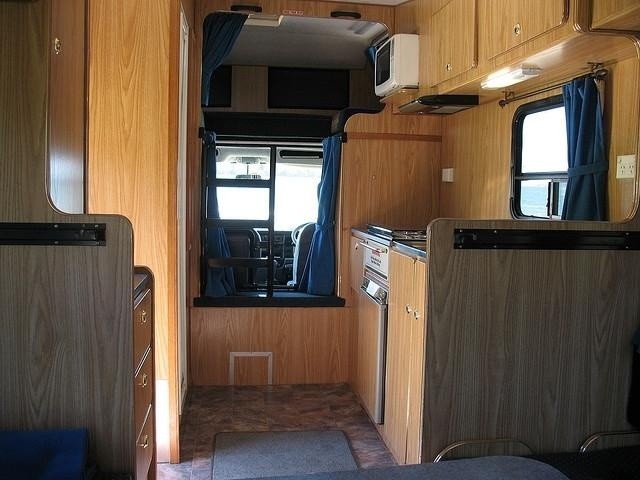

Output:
xmin=366 ymin=223 xmax=427 ymax=242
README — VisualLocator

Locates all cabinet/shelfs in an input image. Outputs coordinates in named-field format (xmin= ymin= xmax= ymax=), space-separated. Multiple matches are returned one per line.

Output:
xmin=421 ymin=0 xmax=478 ymax=88
xmin=45 ymin=0 xmax=87 ymax=215
xmin=134 ymin=284 xmax=156 ymax=480
xmin=380 ymin=251 xmax=426 ymax=466
xmin=480 ymin=0 xmax=569 ymax=63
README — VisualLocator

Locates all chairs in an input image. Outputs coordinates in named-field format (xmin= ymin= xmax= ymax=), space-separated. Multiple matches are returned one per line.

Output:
xmin=223 ymin=226 xmax=263 ymax=292
xmin=287 ymin=220 xmax=317 ymax=291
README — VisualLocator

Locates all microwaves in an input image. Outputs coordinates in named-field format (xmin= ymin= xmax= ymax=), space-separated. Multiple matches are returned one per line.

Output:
xmin=374 ymin=33 xmax=419 ymax=97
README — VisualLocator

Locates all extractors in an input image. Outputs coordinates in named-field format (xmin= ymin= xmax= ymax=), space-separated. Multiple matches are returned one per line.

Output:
xmin=398 ymin=95 xmax=479 ymax=116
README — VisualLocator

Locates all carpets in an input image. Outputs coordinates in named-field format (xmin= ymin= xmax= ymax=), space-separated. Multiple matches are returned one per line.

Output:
xmin=209 ymin=427 xmax=359 ymax=480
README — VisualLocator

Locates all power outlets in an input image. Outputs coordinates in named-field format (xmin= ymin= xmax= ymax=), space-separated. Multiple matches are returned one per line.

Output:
xmin=615 ymin=154 xmax=635 ymax=180
